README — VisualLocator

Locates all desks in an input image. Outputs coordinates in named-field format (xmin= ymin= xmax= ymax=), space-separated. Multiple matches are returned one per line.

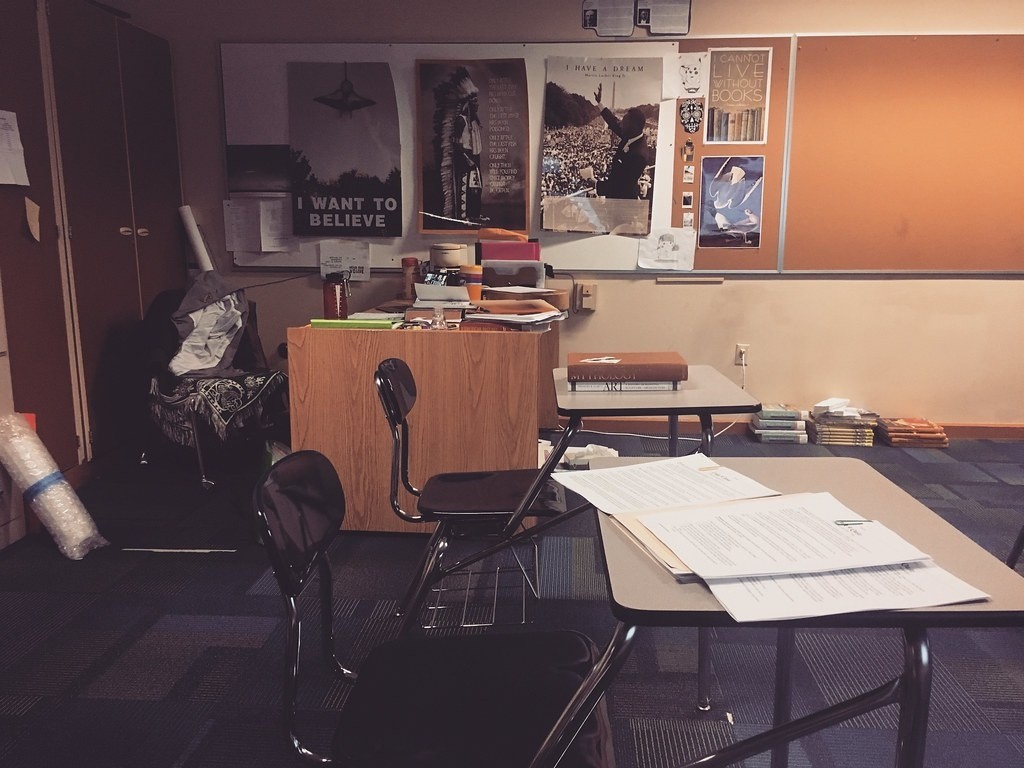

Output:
xmin=527 ymin=455 xmax=1024 ymax=766
xmin=551 ymin=364 xmax=764 ymax=459
xmin=285 ymin=307 xmax=562 ymax=536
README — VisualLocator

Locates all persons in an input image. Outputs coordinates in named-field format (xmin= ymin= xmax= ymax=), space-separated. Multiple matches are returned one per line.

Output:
xmin=585 ymin=10 xmax=595 ymax=27
xmin=543 ymin=114 xmax=653 ymax=209
xmin=638 ymin=11 xmax=649 ymax=24
xmin=585 ymin=81 xmax=649 ymax=199
xmin=433 ymin=66 xmax=490 ymax=224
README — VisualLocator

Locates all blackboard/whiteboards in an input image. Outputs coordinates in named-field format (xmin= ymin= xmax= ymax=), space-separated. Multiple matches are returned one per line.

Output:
xmin=215 ymin=34 xmax=1023 ymax=276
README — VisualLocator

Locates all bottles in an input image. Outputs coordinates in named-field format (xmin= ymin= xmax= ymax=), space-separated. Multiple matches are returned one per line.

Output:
xmin=431 ymin=305 xmax=447 ymax=330
xmin=323 ymin=270 xmax=352 ymax=320
xmin=401 ymin=257 xmax=422 ymax=300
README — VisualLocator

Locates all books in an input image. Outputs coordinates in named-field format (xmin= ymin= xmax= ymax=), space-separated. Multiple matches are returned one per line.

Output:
xmin=568 ymin=351 xmax=688 ymax=393
xmin=310 ymin=238 xmax=567 ymax=332
xmin=750 ymin=405 xmax=949 ymax=449
xmin=551 ymin=453 xmax=992 ymax=623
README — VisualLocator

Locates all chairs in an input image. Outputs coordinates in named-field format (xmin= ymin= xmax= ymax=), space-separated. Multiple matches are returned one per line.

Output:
xmin=148 ymin=286 xmax=287 ymax=493
xmin=372 ymin=357 xmax=570 ymax=644
xmin=253 ymin=449 xmax=607 ymax=768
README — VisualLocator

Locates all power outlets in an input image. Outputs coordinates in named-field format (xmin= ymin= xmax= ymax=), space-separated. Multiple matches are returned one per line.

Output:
xmin=734 ymin=343 xmax=750 ymax=367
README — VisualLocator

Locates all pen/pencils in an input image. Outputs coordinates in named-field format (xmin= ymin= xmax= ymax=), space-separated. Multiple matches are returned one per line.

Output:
xmin=402 ymin=323 xmax=420 ymax=326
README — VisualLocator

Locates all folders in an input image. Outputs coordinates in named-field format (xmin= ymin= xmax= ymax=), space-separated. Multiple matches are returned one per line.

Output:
xmin=311 ymin=318 xmax=404 ymax=330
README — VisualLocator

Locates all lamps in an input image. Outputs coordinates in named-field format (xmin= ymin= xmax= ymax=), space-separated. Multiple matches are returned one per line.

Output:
xmin=309 ymin=59 xmax=378 ymax=122
